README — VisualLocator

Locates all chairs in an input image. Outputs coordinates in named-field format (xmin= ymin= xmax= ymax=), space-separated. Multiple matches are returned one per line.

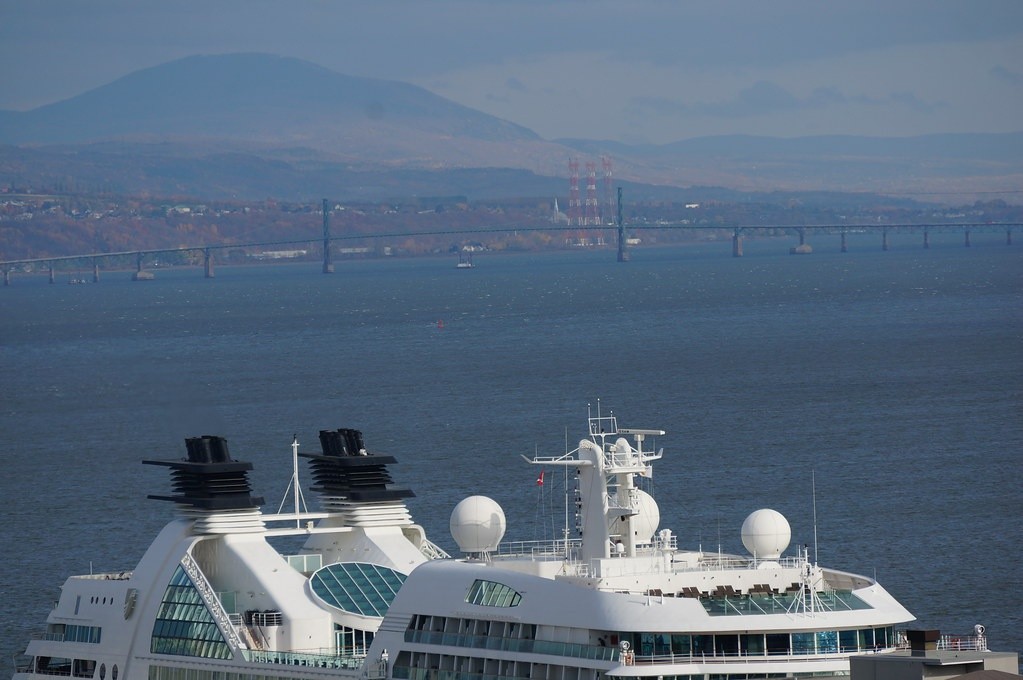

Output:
xmin=647 ymin=589 xmax=664 ymax=597
xmin=749 ymin=583 xmax=776 ymax=599
xmin=714 ymin=584 xmax=739 ymax=601
xmin=682 ymin=586 xmax=703 ymax=600
xmin=787 ymin=583 xmax=811 ymax=594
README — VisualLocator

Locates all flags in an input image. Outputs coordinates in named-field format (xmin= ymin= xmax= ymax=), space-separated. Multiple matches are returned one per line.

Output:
xmin=536 ymin=470 xmax=545 ymax=484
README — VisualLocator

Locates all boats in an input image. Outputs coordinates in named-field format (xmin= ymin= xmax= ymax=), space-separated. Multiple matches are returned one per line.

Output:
xmin=13 ymin=395 xmax=1023 ymax=680
xmin=457 ymin=253 xmax=475 ymax=268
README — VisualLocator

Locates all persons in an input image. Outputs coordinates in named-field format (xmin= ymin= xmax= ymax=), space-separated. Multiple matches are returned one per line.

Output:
xmin=873 ymin=643 xmax=881 ymax=652
xmin=646 ymin=634 xmax=664 ymax=644
xmin=598 ymin=638 xmax=605 ymax=647
